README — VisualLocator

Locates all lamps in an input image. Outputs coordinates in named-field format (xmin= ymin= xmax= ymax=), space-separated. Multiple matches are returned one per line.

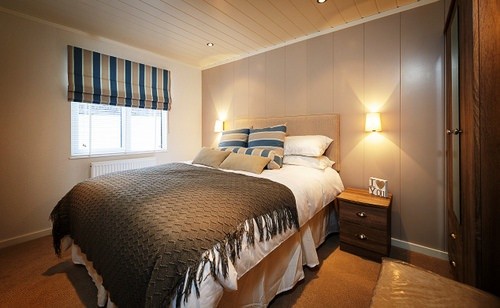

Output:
xmin=214 ymin=119 xmax=223 ymax=132
xmin=364 ymin=109 xmax=381 ymax=134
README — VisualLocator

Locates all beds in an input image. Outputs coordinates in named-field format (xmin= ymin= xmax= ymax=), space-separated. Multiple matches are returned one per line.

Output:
xmin=48 ymin=113 xmax=342 ymax=308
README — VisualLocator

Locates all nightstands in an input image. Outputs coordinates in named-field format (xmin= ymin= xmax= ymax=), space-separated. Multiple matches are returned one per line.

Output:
xmin=337 ymin=188 xmax=393 ymax=261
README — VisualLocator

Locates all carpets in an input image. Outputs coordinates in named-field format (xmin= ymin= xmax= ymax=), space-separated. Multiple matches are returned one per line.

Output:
xmin=268 ymin=240 xmax=383 ymax=308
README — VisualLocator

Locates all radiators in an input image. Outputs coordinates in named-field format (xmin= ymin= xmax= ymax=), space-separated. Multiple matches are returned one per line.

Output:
xmin=91 ymin=156 xmax=158 ymax=178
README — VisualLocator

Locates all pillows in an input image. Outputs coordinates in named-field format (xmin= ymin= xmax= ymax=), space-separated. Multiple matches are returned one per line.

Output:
xmin=191 ymin=123 xmax=335 ymax=174
